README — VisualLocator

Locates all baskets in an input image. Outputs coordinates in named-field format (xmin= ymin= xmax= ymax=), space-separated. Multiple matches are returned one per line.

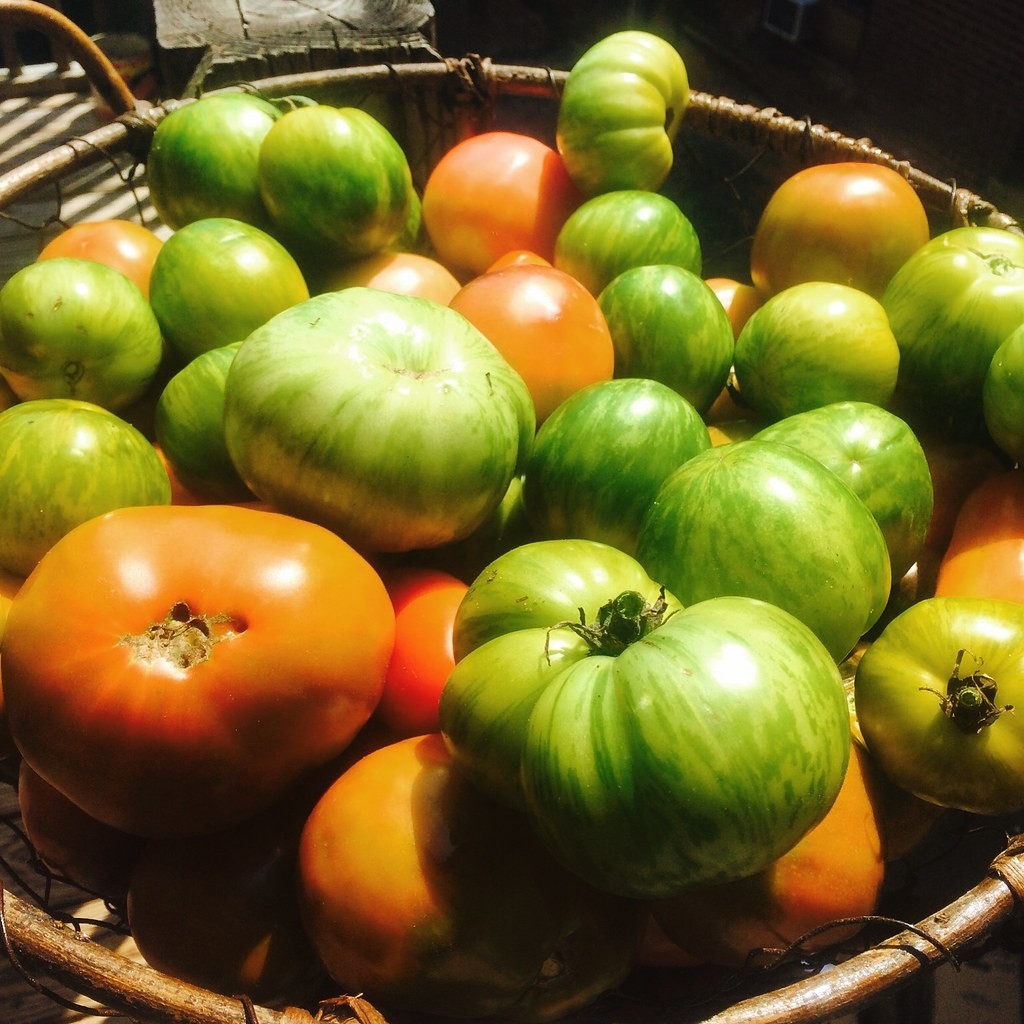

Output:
xmin=1 ymin=62 xmax=1023 ymax=1024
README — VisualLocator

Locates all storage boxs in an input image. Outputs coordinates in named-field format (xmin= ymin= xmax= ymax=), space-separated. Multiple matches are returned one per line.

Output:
xmin=0 ymin=0 xmax=1024 ymax=1022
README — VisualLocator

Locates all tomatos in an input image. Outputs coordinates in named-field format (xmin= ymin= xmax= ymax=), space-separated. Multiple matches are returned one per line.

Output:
xmin=1 ymin=25 xmax=1024 ymax=1018
